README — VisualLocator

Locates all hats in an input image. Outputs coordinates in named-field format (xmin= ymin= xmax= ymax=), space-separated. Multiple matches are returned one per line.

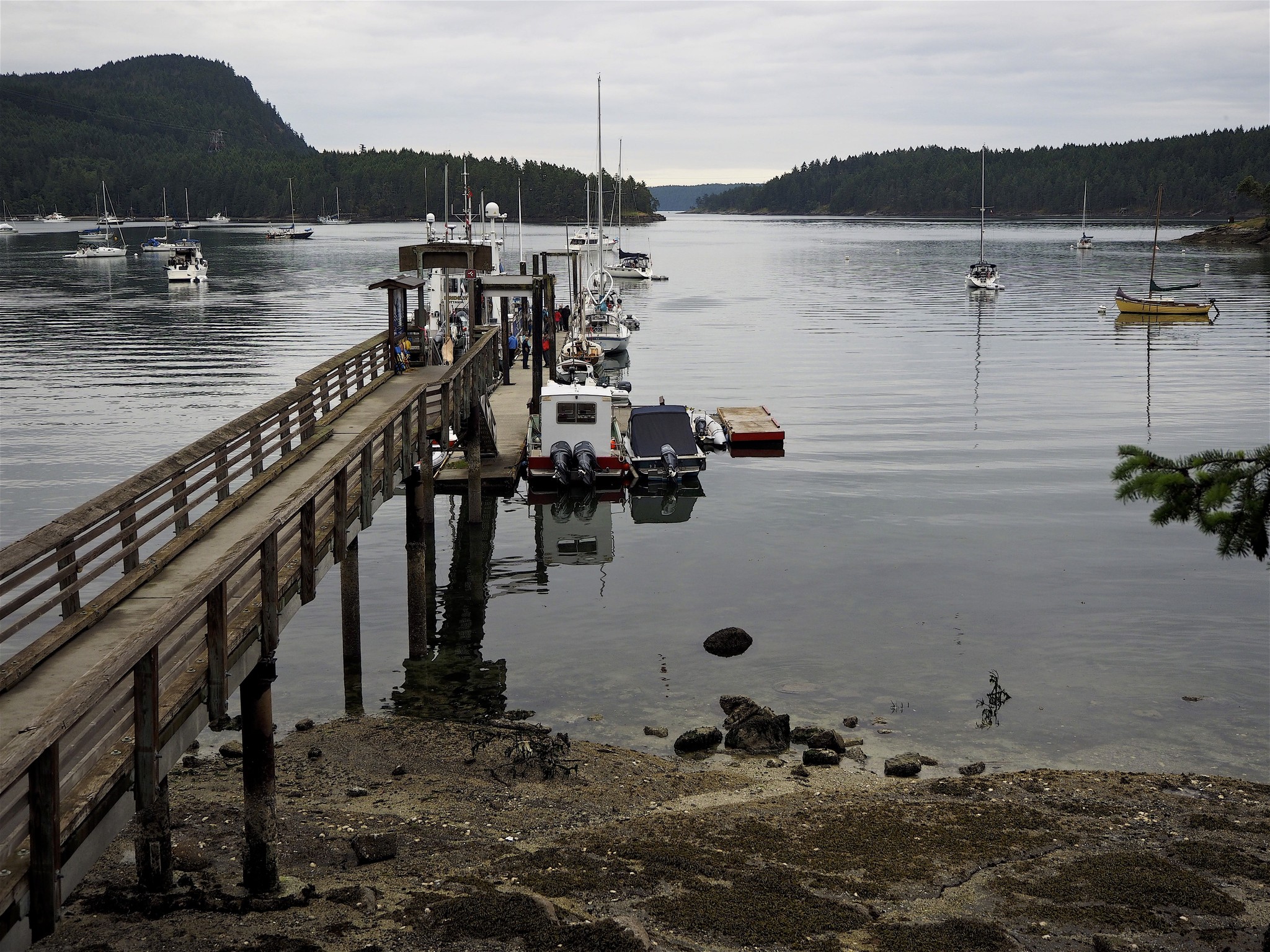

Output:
xmin=555 ymin=308 xmax=558 ymax=311
xmin=565 ymin=305 xmax=569 ymax=308
xmin=544 ymin=308 xmax=548 ymax=311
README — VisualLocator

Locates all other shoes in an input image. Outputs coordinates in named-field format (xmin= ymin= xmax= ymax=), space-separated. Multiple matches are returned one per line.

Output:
xmin=559 ymin=329 xmax=562 ymax=331
xmin=546 ymin=366 xmax=550 ymax=368
xmin=523 ymin=368 xmax=530 ymax=369
xmin=543 ymin=365 xmax=547 ymax=367
xmin=557 ymin=331 xmax=559 ymax=332
xmin=509 ymin=366 xmax=513 ymax=368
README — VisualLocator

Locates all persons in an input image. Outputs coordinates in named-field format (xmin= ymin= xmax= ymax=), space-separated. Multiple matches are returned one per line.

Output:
xmin=558 ymin=305 xmax=564 ymax=331
xmin=561 ymin=305 xmax=571 ymax=332
xmin=522 ymin=331 xmax=531 ymax=366
xmin=508 ymin=332 xmax=518 ymax=369
xmin=542 ymin=332 xmax=550 ymax=368
xmin=542 ymin=307 xmax=549 ymax=334
xmin=521 ymin=337 xmax=531 ymax=369
xmin=555 ymin=308 xmax=562 ymax=333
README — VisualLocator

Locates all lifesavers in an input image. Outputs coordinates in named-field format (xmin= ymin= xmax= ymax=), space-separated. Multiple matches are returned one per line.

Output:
xmin=481 ymin=296 xmax=484 ymax=303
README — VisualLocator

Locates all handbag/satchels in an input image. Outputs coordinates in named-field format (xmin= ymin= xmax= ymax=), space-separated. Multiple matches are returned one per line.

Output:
xmin=561 ymin=314 xmax=567 ymax=319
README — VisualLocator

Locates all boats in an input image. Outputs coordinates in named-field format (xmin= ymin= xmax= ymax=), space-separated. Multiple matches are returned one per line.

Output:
xmin=152 ymin=215 xmax=174 ymax=221
xmin=1072 ymin=233 xmax=1095 ymax=251
xmin=267 ymin=224 xmax=315 ymax=240
xmin=62 ymin=247 xmax=128 ymax=258
xmin=172 ymin=221 xmax=201 ymax=229
xmin=205 ymin=213 xmax=231 ymax=223
xmin=600 ymin=252 xmax=669 ymax=282
xmin=554 ymin=284 xmax=640 ymax=382
xmin=316 ymin=214 xmax=353 ymax=224
xmin=564 ymin=224 xmax=619 ymax=251
xmin=160 ymin=239 xmax=208 ymax=283
xmin=33 ymin=212 xmax=72 ymax=223
xmin=140 ymin=236 xmax=188 ymax=252
xmin=1107 ymin=287 xmax=1218 ymax=316
xmin=962 ymin=259 xmax=1008 ymax=291
xmin=524 ymin=378 xmax=788 ymax=504
xmin=78 ymin=230 xmax=115 ymax=239
xmin=96 ymin=214 xmax=136 ymax=225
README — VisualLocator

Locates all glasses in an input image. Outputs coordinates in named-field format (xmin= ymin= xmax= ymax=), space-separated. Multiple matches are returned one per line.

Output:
xmin=527 ymin=339 xmax=528 ymax=339
xmin=526 ymin=334 xmax=528 ymax=334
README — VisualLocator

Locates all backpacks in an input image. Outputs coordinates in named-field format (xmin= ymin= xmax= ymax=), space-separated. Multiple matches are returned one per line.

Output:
xmin=544 ymin=311 xmax=549 ymax=323
